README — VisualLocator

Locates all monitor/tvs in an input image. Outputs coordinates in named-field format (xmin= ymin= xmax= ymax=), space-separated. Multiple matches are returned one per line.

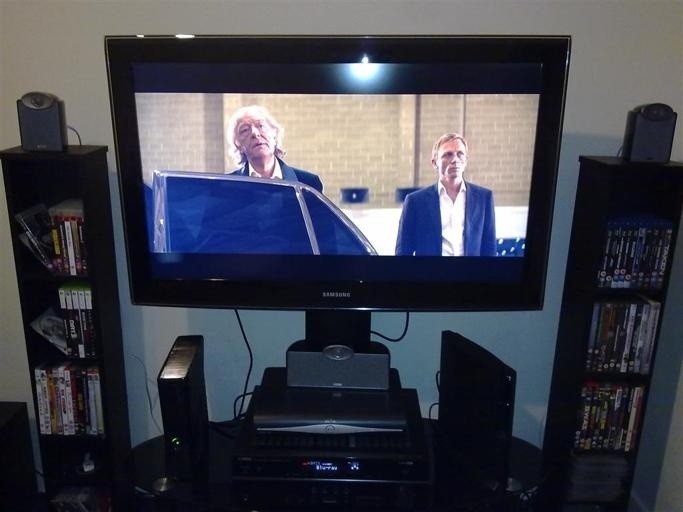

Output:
xmin=102 ymin=34 xmax=573 ymax=388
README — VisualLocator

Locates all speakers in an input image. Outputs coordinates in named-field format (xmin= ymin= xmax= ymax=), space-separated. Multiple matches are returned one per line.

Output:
xmin=620 ymin=103 xmax=679 ymax=164
xmin=438 ymin=330 xmax=520 ymax=497
xmin=157 ymin=334 xmax=213 ymax=473
xmin=15 ymin=90 xmax=69 ymax=153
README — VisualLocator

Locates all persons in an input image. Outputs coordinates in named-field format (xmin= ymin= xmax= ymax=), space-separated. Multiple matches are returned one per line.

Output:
xmin=394 ymin=132 xmax=497 ymax=256
xmin=221 ymin=104 xmax=323 ymax=194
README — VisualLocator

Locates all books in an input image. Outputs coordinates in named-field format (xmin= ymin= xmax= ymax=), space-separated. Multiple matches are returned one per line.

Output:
xmin=574 ymin=216 xmax=674 ymax=453
xmin=14 ymin=197 xmax=105 ymax=436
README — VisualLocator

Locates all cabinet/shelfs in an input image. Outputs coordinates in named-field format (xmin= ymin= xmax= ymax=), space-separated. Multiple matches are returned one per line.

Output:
xmin=0 ymin=144 xmax=132 ymax=512
xmin=543 ymin=156 xmax=683 ymax=512
xmin=118 ymin=418 xmax=552 ymax=512
